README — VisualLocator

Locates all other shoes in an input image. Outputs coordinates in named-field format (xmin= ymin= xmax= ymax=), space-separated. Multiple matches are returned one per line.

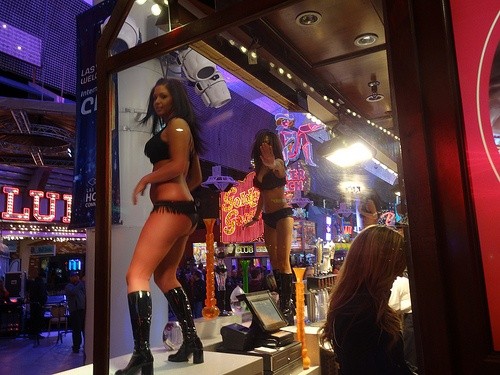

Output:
xmin=73 ymin=348 xmax=79 ymax=352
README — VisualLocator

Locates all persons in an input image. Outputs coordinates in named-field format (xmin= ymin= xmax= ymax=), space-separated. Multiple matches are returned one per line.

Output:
xmin=0 ymin=273 xmax=86 ymax=353
xmin=176 ymin=264 xmax=280 ymax=316
xmin=243 ymin=130 xmax=297 ymax=326
xmin=114 ymin=77 xmax=204 ymax=375
xmin=320 ymin=225 xmax=418 ymax=375
xmin=359 ymin=188 xmax=377 ymax=227
xmin=295 ymin=260 xmax=342 ymax=280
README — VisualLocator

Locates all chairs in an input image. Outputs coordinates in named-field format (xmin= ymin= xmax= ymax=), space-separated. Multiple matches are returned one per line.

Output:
xmin=47 ymin=300 xmax=69 ymax=334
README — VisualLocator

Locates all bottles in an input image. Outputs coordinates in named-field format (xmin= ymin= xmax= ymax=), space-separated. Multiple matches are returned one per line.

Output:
xmin=162 ymin=321 xmax=183 ymax=350
xmin=230 ymin=284 xmax=246 ymax=314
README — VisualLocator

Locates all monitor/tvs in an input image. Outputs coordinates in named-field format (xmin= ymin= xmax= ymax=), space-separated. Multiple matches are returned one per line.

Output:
xmin=242 ymin=289 xmax=290 ymax=333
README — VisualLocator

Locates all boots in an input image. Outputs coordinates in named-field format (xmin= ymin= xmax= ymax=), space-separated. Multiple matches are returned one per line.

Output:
xmin=114 ymin=290 xmax=154 ymax=375
xmin=164 ymin=287 xmax=204 ymax=364
xmin=276 ymin=273 xmax=294 ymax=326
xmin=273 ymin=269 xmax=296 ymax=316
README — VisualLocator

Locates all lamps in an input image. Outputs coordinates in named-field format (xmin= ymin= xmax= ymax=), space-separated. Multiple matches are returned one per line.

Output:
xmin=194 ymin=70 xmax=231 ymax=108
xmin=100 ymin=15 xmax=140 ymax=55
xmin=177 ymin=47 xmax=217 ymax=81
